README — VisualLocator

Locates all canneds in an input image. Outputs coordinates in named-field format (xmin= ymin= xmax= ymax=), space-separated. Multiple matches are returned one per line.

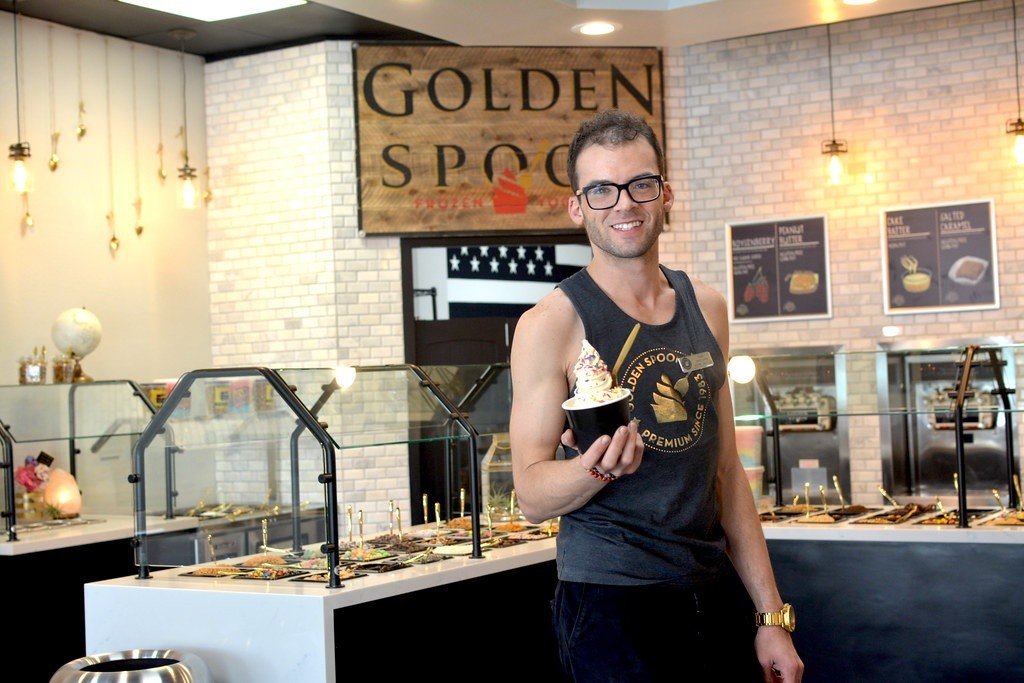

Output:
xmin=138 ymin=376 xmax=274 ymax=419
xmin=18 ymin=354 xmax=75 ymax=384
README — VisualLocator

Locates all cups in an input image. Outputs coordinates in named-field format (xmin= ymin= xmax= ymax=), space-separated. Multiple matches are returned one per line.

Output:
xmin=561 ymin=388 xmax=631 ymax=455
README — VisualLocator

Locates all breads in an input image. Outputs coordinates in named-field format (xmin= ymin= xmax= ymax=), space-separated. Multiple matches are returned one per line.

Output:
xmin=957 ymin=260 xmax=984 ymax=279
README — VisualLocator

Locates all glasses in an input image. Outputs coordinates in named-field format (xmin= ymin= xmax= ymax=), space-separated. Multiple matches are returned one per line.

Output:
xmin=575 ymin=174 xmax=664 ymax=211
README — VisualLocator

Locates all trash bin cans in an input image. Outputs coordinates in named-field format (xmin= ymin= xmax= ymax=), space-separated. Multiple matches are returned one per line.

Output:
xmin=51 ymin=649 xmax=213 ymax=683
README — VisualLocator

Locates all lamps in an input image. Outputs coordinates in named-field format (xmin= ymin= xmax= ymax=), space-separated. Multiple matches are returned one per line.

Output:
xmin=728 ymin=355 xmax=756 ymax=382
xmin=46 ymin=469 xmax=83 ymax=521
xmin=7 ymin=6 xmax=197 ymax=255
xmin=1007 ymin=0 xmax=1024 ymax=166
xmin=284 ymin=367 xmax=355 ymax=557
xmin=822 ymin=25 xmax=848 ymax=186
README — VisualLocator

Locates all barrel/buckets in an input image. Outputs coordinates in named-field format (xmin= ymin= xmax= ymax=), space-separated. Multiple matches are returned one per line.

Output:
xmin=731 ymin=425 xmax=765 ymax=519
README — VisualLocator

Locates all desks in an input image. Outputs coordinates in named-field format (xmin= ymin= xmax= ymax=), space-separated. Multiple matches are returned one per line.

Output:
xmin=0 ymin=505 xmax=1024 ymax=683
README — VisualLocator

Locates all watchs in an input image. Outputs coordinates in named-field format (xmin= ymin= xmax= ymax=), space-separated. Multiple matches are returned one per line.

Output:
xmin=755 ymin=604 xmax=796 ymax=633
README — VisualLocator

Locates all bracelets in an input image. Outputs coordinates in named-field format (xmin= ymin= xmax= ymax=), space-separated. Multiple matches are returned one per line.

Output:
xmin=588 ymin=467 xmax=619 ymax=481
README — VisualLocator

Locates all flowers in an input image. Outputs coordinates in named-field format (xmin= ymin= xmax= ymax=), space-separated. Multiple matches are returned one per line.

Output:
xmin=15 ymin=453 xmax=54 ymax=492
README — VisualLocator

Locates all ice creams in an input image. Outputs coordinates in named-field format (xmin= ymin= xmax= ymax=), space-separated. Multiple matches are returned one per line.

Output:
xmin=573 ymin=339 xmax=622 ymax=407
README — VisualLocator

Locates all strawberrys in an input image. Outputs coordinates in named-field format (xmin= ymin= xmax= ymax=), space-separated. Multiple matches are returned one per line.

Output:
xmin=744 ymin=280 xmax=769 ymax=303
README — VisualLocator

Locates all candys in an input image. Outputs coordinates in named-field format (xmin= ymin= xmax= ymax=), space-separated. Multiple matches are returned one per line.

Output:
xmin=248 ymin=546 xmax=390 ymax=578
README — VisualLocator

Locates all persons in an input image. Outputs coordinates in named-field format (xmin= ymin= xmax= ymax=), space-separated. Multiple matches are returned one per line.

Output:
xmin=510 ymin=111 xmax=804 ymax=683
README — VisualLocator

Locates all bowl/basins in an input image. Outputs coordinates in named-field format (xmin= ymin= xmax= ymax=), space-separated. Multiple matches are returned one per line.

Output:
xmin=948 ymin=254 xmax=989 ymax=286
xmin=901 ymin=267 xmax=932 ymax=295
